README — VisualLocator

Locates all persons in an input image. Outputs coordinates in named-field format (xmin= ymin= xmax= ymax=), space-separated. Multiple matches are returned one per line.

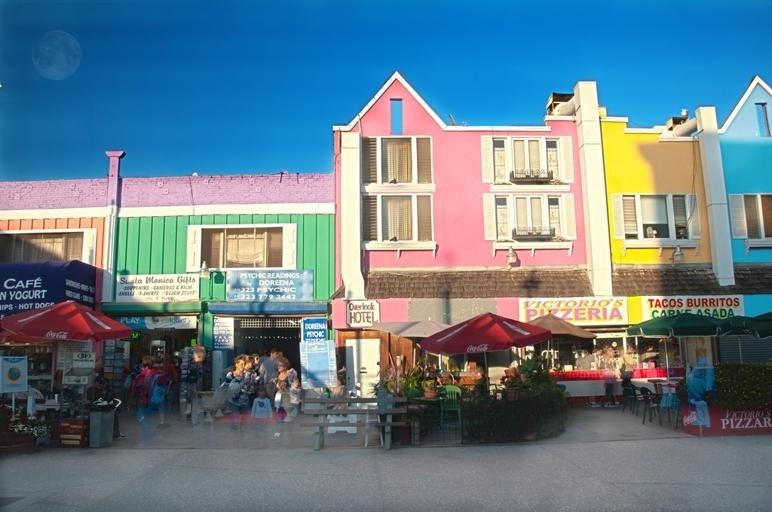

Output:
xmin=469 ymin=366 xmax=488 ymax=398
xmin=573 ymin=344 xmax=638 ymax=408
xmin=92 ymin=346 xmax=302 ymax=449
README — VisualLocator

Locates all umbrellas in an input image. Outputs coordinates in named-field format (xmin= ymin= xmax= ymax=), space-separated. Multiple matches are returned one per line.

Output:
xmin=526 ymin=310 xmax=598 ymax=367
xmin=0 ymin=298 xmax=135 ymax=391
xmin=420 ymin=311 xmax=552 ymax=391
xmin=753 ymin=311 xmax=772 ymax=337
xmin=722 ymin=314 xmax=754 ymax=363
xmin=628 ymin=311 xmax=731 ymax=374
xmin=372 ymin=316 xmax=451 ymax=339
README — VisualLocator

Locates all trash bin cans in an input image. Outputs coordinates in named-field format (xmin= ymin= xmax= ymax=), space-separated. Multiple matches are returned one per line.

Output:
xmin=88 ymin=404 xmax=115 ymax=447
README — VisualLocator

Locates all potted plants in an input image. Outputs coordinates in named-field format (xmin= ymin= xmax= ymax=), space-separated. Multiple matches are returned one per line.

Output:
xmin=504 ymin=379 xmax=520 ymax=402
xmin=424 ymin=380 xmax=439 ymax=399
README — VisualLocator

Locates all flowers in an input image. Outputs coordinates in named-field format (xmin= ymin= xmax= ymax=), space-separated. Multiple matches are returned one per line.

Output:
xmin=0 ymin=404 xmax=52 ymax=445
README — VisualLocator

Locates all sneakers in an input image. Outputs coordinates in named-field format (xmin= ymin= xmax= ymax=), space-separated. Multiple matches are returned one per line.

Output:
xmin=157 ymin=422 xmax=171 ymax=429
xmin=116 ymin=433 xmax=127 ymax=440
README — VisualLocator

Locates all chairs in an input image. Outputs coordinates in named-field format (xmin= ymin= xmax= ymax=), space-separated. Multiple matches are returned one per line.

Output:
xmin=439 ymin=385 xmax=464 ymax=443
xmin=621 ymin=378 xmax=670 ymax=427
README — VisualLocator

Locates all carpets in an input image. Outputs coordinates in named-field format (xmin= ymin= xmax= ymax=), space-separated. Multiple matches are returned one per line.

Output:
xmin=209 ymin=408 xmax=286 ymax=423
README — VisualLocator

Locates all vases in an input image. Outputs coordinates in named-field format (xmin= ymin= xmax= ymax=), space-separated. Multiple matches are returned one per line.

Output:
xmin=0 ymin=432 xmax=36 ymax=450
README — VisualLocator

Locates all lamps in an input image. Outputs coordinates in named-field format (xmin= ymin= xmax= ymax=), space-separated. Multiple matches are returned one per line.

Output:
xmin=670 ymin=246 xmax=684 ymax=265
xmin=506 ymin=245 xmax=518 ymax=264
xmin=200 ymin=260 xmax=211 ymax=279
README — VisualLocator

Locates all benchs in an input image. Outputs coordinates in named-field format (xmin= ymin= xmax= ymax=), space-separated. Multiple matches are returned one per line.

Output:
xmin=300 ymin=398 xmax=408 ymax=451
